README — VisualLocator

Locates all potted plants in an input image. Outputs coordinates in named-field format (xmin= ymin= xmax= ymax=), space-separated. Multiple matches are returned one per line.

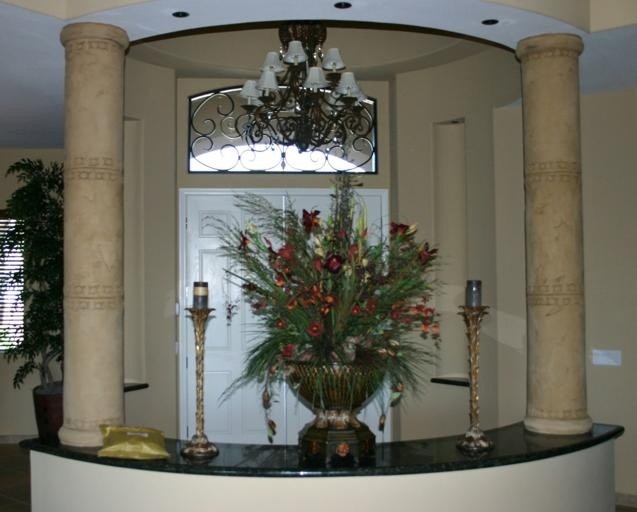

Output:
xmin=0 ymin=154 xmax=63 ymax=437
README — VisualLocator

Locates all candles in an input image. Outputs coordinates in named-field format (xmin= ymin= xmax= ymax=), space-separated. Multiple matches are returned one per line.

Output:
xmin=465 ymin=279 xmax=482 ymax=307
xmin=192 ymin=280 xmax=209 ymax=309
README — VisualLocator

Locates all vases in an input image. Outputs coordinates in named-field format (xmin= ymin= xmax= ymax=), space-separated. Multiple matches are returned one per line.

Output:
xmin=284 ymin=358 xmax=379 ymax=468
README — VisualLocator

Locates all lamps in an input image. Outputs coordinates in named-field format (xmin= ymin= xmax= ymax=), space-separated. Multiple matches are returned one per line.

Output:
xmin=233 ymin=22 xmax=375 ymax=153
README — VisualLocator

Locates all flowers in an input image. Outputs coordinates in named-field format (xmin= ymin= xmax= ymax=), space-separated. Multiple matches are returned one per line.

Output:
xmin=213 ymin=168 xmax=443 ymax=446
